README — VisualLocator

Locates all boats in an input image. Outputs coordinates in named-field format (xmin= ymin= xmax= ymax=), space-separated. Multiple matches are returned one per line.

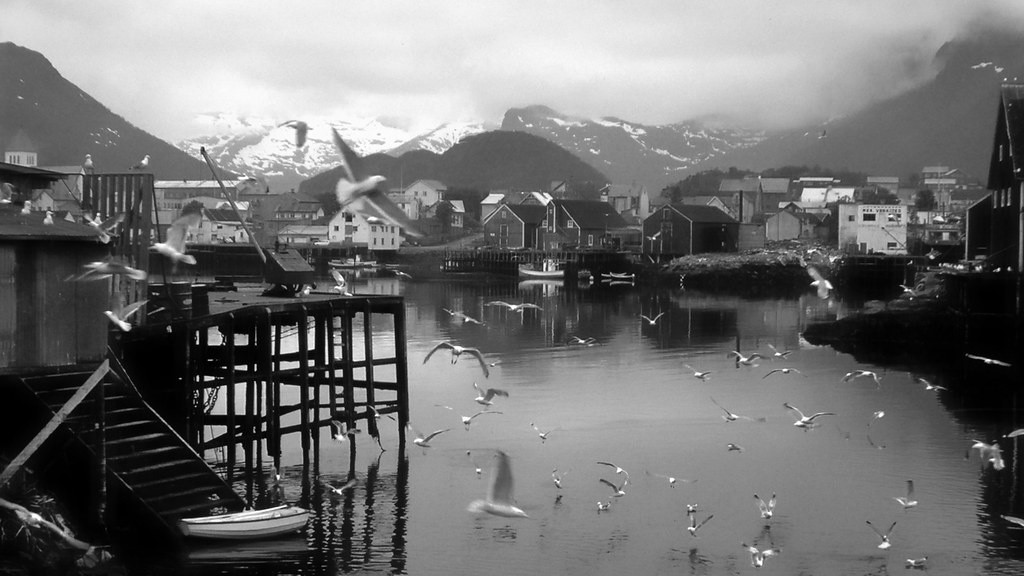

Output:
xmin=176 ymin=502 xmax=312 ymax=540
xmin=182 ymin=535 xmax=319 ymax=571
xmin=609 ymin=271 xmax=635 ymax=281
xmin=600 ymin=272 xmax=628 ymax=279
xmin=517 ymin=262 xmax=565 ymax=279
xmin=327 ymin=258 xmax=377 ymax=269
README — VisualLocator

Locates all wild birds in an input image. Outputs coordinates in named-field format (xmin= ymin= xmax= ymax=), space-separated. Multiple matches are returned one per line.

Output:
xmin=20 ymin=200 xmax=32 ymax=215
xmin=405 ymin=422 xmax=450 ymax=455
xmin=806 ymin=263 xmax=834 ymax=299
xmin=465 ymin=447 xmax=530 ymax=518
xmin=566 ymin=335 xmax=602 ymax=348
xmin=483 ymin=300 xmax=544 ymax=313
xmin=966 ymin=353 xmax=1012 ymax=369
xmin=684 ymin=344 xmax=886 ymax=451
xmin=552 ymin=467 xmax=571 ymax=489
xmin=434 ymin=404 xmax=504 ymax=431
xmin=277 ymin=119 xmax=314 ymax=148
xmin=318 ymin=478 xmax=357 ymax=496
xmin=129 ymin=155 xmax=152 ymax=172
xmin=368 ymin=405 xmax=397 ymax=422
xmin=421 ymin=342 xmax=490 ymax=379
xmin=83 ymin=212 xmax=126 ymax=244
xmin=0 ymin=183 xmax=15 ymax=204
xmin=452 ymin=310 xmax=485 ymax=324
xmin=147 ymin=213 xmax=198 ymax=266
xmin=918 ymin=378 xmax=948 ymax=392
xmin=65 ymin=257 xmax=148 ymax=283
xmin=530 ymin=422 xmax=561 ymax=444
xmin=328 ymin=265 xmax=355 ymax=297
xmin=43 ymin=210 xmax=54 ymax=224
xmin=331 ymin=420 xmax=361 ymax=442
xmin=330 ymin=129 xmax=428 ymax=239
xmin=102 ymin=292 xmax=151 ymax=332
xmin=473 ymin=380 xmax=509 ymax=407
xmin=84 ymin=154 xmax=96 ymax=174
xmin=0 ymin=498 xmax=91 ymax=552
xmin=639 ymin=312 xmax=665 ymax=325
xmin=965 ymin=438 xmax=1006 ymax=470
xmin=642 ymin=466 xmax=930 ymax=568
xmin=597 ymin=461 xmax=630 ymax=510
